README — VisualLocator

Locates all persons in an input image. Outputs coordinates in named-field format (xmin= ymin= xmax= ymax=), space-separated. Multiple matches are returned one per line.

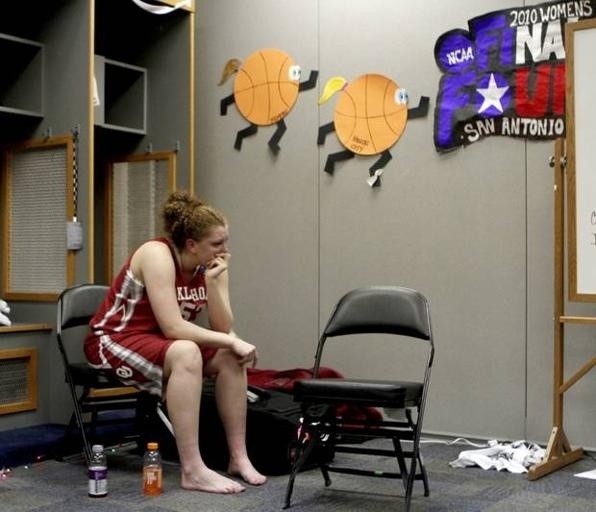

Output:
xmin=81 ymin=191 xmax=268 ymax=494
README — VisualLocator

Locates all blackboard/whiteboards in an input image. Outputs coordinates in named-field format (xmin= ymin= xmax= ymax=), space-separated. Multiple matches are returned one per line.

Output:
xmin=564 ymin=17 xmax=596 ymax=302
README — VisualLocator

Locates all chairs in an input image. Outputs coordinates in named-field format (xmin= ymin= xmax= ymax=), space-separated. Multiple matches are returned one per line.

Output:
xmin=282 ymin=285 xmax=434 ymax=512
xmin=55 ymin=283 xmax=144 ymax=475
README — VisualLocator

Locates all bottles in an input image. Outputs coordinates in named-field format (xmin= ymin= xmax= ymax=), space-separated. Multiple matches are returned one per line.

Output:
xmin=86 ymin=447 xmax=110 ymax=499
xmin=140 ymin=444 xmax=162 ymax=497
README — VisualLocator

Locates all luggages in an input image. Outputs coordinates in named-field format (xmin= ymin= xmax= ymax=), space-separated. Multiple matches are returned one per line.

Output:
xmin=134 ymin=381 xmax=337 ymax=477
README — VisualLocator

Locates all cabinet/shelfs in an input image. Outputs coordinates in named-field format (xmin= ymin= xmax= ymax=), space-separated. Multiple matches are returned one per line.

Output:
xmin=0 ymin=1 xmax=196 ymax=470
